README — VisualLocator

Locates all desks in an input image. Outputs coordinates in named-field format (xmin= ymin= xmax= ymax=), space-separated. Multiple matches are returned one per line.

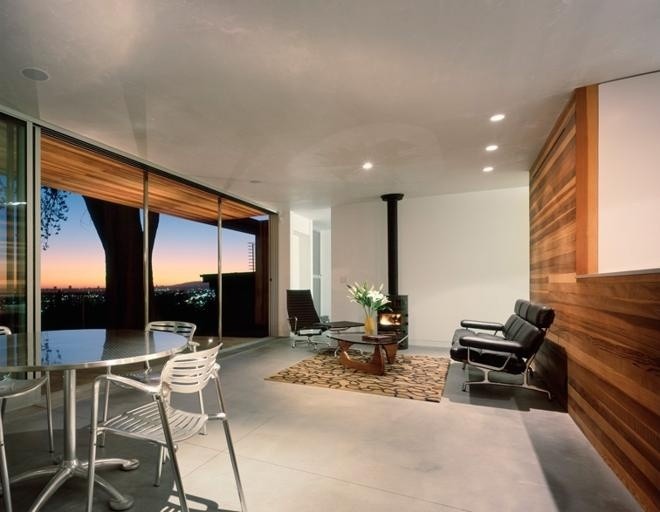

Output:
xmin=312 ymin=319 xmax=364 ymax=358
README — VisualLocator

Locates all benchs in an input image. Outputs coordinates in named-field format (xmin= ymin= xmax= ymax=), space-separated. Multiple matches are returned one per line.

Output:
xmin=450 ymin=298 xmax=555 ymax=402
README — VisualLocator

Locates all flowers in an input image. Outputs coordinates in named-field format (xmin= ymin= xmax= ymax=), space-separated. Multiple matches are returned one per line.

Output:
xmin=347 ymin=280 xmax=392 ymax=317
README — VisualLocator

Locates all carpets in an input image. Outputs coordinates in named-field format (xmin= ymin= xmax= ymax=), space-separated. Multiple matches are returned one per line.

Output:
xmin=264 ymin=348 xmax=453 ymax=404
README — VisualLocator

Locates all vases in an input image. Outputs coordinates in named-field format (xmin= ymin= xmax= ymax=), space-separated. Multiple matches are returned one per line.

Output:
xmin=364 ymin=314 xmax=375 ymax=337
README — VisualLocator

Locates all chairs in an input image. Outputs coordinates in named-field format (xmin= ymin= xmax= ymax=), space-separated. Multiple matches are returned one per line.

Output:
xmin=286 ymin=287 xmax=331 ymax=351
